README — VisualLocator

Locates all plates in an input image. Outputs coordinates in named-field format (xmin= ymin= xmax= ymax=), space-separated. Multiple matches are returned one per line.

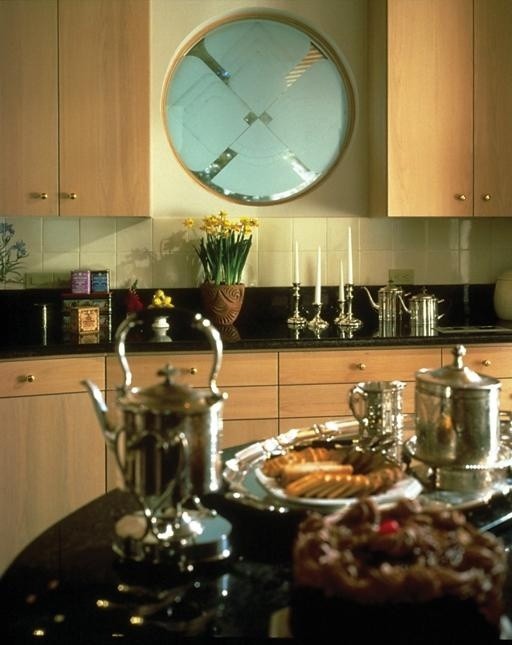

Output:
xmin=256 ymin=453 xmax=422 ymax=506
xmin=402 ymin=436 xmax=510 ymax=469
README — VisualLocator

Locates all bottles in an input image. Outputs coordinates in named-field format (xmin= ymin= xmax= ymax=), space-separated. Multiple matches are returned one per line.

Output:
xmin=414 ymin=345 xmax=500 ymax=467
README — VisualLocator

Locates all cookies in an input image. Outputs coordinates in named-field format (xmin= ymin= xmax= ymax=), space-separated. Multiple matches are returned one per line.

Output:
xmin=260 ymin=446 xmax=403 ymax=499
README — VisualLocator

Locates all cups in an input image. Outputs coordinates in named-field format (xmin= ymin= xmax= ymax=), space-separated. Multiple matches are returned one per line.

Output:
xmin=348 ymin=380 xmax=405 ymax=435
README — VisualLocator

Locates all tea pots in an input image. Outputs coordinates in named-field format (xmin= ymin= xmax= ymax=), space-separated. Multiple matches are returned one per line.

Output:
xmin=78 ymin=306 xmax=225 ymax=502
xmin=363 ymin=280 xmax=403 ymax=322
xmin=397 ymin=289 xmax=447 ymax=324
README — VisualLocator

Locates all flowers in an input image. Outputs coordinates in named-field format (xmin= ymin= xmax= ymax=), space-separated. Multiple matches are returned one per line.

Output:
xmin=183 ymin=211 xmax=259 ymax=285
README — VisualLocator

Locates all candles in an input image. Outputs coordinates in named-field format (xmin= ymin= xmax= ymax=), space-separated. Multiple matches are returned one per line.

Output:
xmin=294 ymin=241 xmax=299 ymax=283
xmin=339 ymin=258 xmax=345 ymax=301
xmin=315 ymin=247 xmax=322 ymax=304
xmin=347 ymin=226 xmax=353 ymax=284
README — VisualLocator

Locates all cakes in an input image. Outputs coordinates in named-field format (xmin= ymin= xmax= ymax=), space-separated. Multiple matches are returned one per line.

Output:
xmin=291 ymin=497 xmax=510 ymax=644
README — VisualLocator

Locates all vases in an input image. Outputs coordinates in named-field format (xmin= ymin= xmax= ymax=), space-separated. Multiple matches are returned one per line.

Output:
xmin=200 ymin=285 xmax=244 ymax=340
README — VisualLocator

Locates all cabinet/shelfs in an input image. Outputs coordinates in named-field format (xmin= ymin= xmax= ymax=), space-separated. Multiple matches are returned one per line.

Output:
xmin=279 ymin=345 xmax=442 ymax=436
xmin=106 ymin=350 xmax=279 ymax=492
xmin=366 ymin=1 xmax=512 ymax=217
xmin=442 ymin=342 xmax=512 ymax=416
xmin=0 ymin=1 xmax=152 ymax=218
xmin=0 ymin=354 xmax=108 ymax=574
